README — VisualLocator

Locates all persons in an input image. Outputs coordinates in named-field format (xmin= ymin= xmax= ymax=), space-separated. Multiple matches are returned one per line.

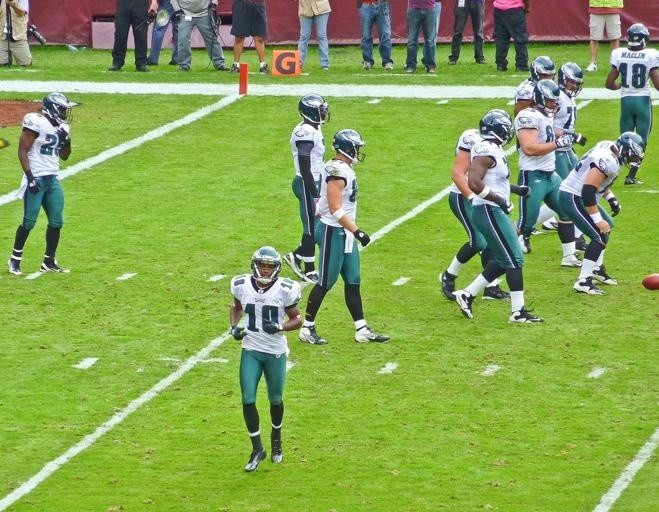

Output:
xmin=230 ymin=244 xmax=303 ymax=473
xmin=403 ymin=0 xmax=439 ymax=74
xmin=493 ymin=0 xmax=532 ymax=71
xmin=7 ymin=92 xmax=83 ymax=274
xmin=605 ymin=23 xmax=658 ymax=185
xmin=297 ymin=129 xmax=390 ymax=346
xmin=230 ymin=0 xmax=272 ymax=74
xmin=108 ymin=0 xmax=159 ymax=71
xmin=586 ymin=0 xmax=625 ymax=71
xmin=297 ymin=0 xmax=333 ymax=69
xmin=444 ymin=0 xmax=490 ymax=65
xmin=283 ymin=92 xmax=330 ymax=284
xmin=438 ymin=55 xmax=648 ymax=322
xmin=0 ymin=0 xmax=32 ymax=66
xmin=170 ymin=0 xmax=230 ymax=70
xmin=146 ymin=0 xmax=178 ymax=64
xmin=405 ymin=0 xmax=442 ymax=68
xmin=357 ymin=0 xmax=394 ymax=70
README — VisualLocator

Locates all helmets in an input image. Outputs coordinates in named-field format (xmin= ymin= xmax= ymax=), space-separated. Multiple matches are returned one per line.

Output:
xmin=479 ymin=55 xmax=584 ymax=145
xmin=251 ymin=245 xmax=283 ymax=285
xmin=299 ymin=92 xmax=331 ymax=124
xmin=625 ymin=23 xmax=649 ymax=51
xmin=613 ymin=131 xmax=645 ymax=169
xmin=333 ymin=128 xmax=368 ymax=163
xmin=42 ymin=93 xmax=81 ymax=125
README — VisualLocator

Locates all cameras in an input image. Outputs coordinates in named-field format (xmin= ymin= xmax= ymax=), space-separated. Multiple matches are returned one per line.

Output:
xmin=24 ymin=22 xmax=48 ymax=49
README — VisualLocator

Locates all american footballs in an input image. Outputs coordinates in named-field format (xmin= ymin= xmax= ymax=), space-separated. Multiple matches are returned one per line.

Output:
xmin=641 ymin=274 xmax=659 ymax=290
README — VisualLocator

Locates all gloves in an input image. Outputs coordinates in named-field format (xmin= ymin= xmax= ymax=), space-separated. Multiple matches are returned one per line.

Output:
xmin=57 ymin=127 xmax=71 ymax=146
xmin=25 ymin=170 xmax=41 ymax=195
xmin=262 ymin=319 xmax=281 ymax=335
xmin=497 ymin=197 xmax=514 ymax=215
xmin=516 ymin=184 xmax=533 ymax=199
xmin=231 ymin=326 xmax=246 ymax=340
xmin=354 ymin=230 xmax=370 ymax=248
xmin=607 ymin=197 xmax=620 ymax=217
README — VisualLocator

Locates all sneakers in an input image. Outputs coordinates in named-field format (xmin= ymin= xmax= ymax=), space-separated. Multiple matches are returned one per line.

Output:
xmin=440 ymin=217 xmax=619 ymax=324
xmin=586 ymin=65 xmax=598 ymax=74
xmin=244 ymin=449 xmax=266 ymax=472
xmin=272 ymin=440 xmax=282 ymax=463
xmin=353 ymin=331 xmax=390 ymax=343
xmin=41 ymin=260 xmax=69 ymax=274
xmin=625 ymin=177 xmax=644 ymax=184
xmin=298 ymin=324 xmax=328 ymax=345
xmin=284 ymin=253 xmax=319 ymax=284
xmin=7 ymin=259 xmax=22 ymax=275
